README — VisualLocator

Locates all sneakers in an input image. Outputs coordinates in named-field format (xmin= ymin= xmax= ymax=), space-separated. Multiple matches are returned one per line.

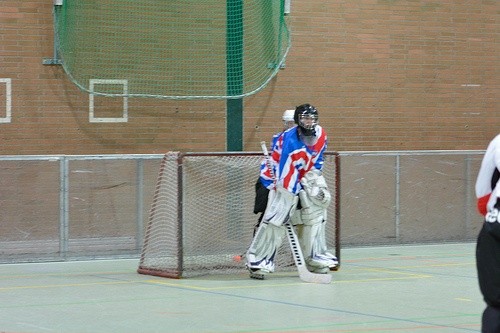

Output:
xmin=244 ymin=250 xmax=339 ymax=279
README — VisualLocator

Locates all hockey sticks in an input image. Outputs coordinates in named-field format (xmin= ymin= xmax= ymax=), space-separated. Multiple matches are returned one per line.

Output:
xmin=260 ymin=141 xmax=332 ymax=285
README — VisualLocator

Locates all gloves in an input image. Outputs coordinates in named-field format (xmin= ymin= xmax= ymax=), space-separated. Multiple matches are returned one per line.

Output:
xmin=301 ymin=172 xmax=331 ymax=208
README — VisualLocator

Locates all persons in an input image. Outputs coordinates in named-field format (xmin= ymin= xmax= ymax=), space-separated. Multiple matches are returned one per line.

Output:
xmin=475 ymin=134 xmax=500 ymax=333
xmin=253 ymin=110 xmax=339 ymax=273
xmin=245 ymin=103 xmax=331 ymax=280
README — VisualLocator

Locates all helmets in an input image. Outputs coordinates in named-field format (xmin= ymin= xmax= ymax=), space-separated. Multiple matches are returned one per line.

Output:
xmin=282 ymin=107 xmax=297 ymax=121
xmin=294 ymin=103 xmax=318 ymax=137
